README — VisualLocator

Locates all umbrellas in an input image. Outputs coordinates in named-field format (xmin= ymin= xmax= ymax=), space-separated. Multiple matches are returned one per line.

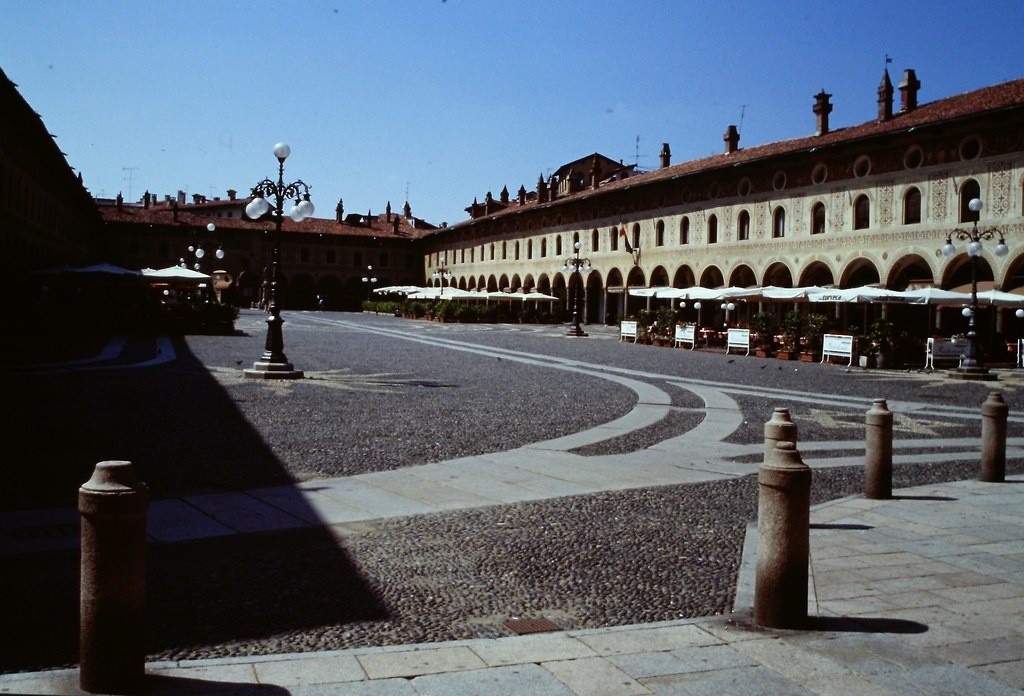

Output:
xmin=372 ymin=285 xmax=559 ymax=313
xmin=629 ymin=283 xmax=1024 ymax=345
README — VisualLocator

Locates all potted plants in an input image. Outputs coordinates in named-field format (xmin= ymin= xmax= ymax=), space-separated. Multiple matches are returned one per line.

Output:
xmin=801 ymin=313 xmax=828 ymax=361
xmin=857 ymin=336 xmax=873 ymax=368
xmin=867 ymin=318 xmax=894 ymax=369
xmin=751 ymin=312 xmax=775 ymax=357
xmin=663 ymin=310 xmax=677 ymax=348
xmin=636 ymin=310 xmax=650 ymax=345
xmin=651 ymin=306 xmax=667 ymax=346
xmin=776 ymin=310 xmax=805 ymax=360
xmin=359 ymin=301 xmax=549 ymax=325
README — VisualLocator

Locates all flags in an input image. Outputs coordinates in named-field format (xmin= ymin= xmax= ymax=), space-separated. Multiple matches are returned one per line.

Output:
xmin=619 ymin=217 xmax=626 ymax=237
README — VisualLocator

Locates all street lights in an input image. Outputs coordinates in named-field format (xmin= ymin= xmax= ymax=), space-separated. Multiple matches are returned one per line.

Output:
xmin=942 ymin=199 xmax=1009 ymax=381
xmin=161 ymin=221 xmax=227 ymax=305
xmin=431 ymin=257 xmax=453 ymax=295
xmin=241 ymin=142 xmax=317 ymax=379
xmin=561 ymin=242 xmax=592 ymax=337
xmin=361 ymin=264 xmax=378 ymax=303
